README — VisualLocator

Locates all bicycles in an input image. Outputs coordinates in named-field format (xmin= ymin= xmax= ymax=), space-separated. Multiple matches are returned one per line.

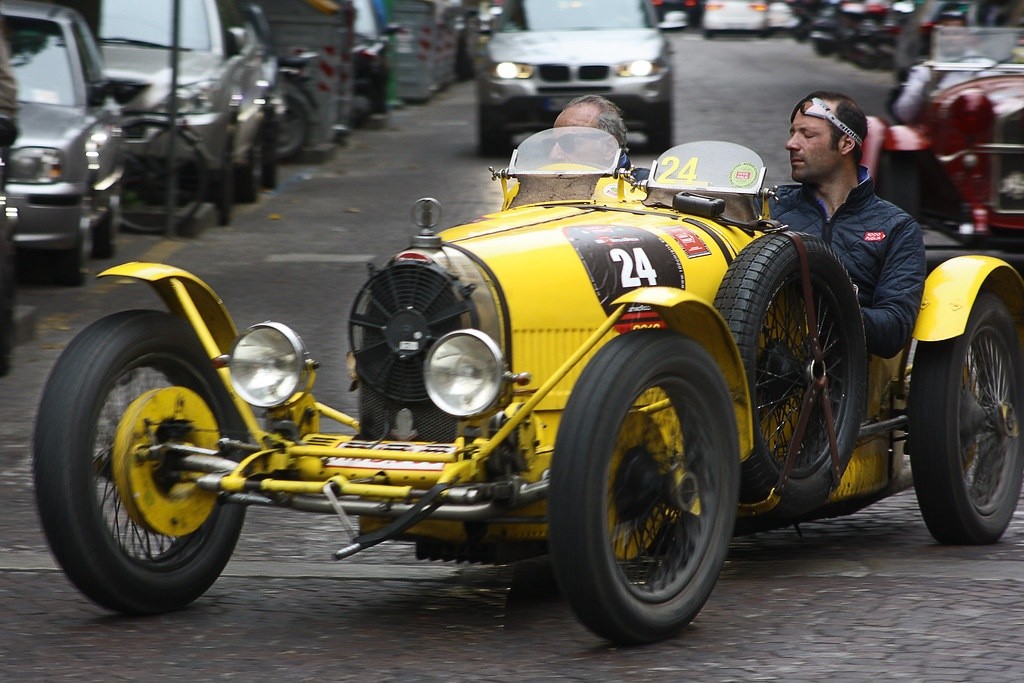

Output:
xmin=110 ymin=110 xmax=207 ymax=234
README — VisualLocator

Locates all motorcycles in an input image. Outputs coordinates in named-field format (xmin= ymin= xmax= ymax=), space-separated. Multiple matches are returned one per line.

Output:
xmin=267 ymin=51 xmax=321 ymax=157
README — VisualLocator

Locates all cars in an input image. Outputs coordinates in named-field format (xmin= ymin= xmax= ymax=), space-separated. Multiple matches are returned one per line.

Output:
xmin=478 ymin=0 xmax=686 ymax=153
xmin=28 ymin=125 xmax=1024 ymax=643
xmin=0 ymin=0 xmax=124 ymax=286
xmin=349 ymin=3 xmax=404 ymax=117
xmin=53 ymin=0 xmax=281 ymax=226
xmin=655 ymin=1 xmax=1006 ymax=65
xmin=861 ymin=24 xmax=1023 ymax=254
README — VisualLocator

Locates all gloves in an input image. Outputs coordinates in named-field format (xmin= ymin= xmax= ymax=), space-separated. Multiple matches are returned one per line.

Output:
xmin=0 ymin=117 xmax=17 ymax=147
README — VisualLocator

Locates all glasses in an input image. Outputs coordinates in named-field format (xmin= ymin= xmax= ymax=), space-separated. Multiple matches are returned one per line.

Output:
xmin=542 ymin=133 xmax=601 ymax=153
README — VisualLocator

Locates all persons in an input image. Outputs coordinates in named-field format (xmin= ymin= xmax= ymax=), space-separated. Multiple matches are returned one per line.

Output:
xmin=754 ymin=91 xmax=926 ymax=410
xmin=887 ymin=10 xmax=989 ymax=123
xmin=550 ymin=95 xmax=651 ymax=183
